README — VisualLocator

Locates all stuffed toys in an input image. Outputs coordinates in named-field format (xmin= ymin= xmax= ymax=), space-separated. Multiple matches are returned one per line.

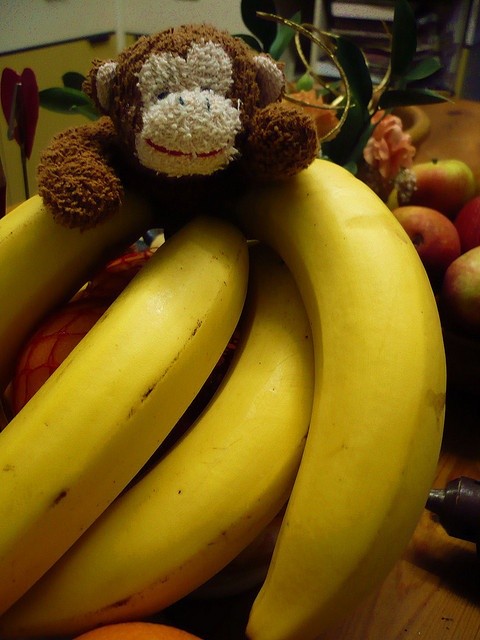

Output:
xmin=38 ymin=24 xmax=317 ymax=232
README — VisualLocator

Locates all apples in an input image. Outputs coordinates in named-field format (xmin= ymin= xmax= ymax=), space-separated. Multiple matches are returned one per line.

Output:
xmin=442 ymin=245 xmax=479 ymax=327
xmin=398 ymin=159 xmax=475 ymax=214
xmin=391 ymin=205 xmax=462 ymax=284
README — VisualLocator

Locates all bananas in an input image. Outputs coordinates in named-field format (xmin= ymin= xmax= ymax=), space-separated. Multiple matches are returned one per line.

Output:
xmin=0 ymin=158 xmax=449 ymax=636
